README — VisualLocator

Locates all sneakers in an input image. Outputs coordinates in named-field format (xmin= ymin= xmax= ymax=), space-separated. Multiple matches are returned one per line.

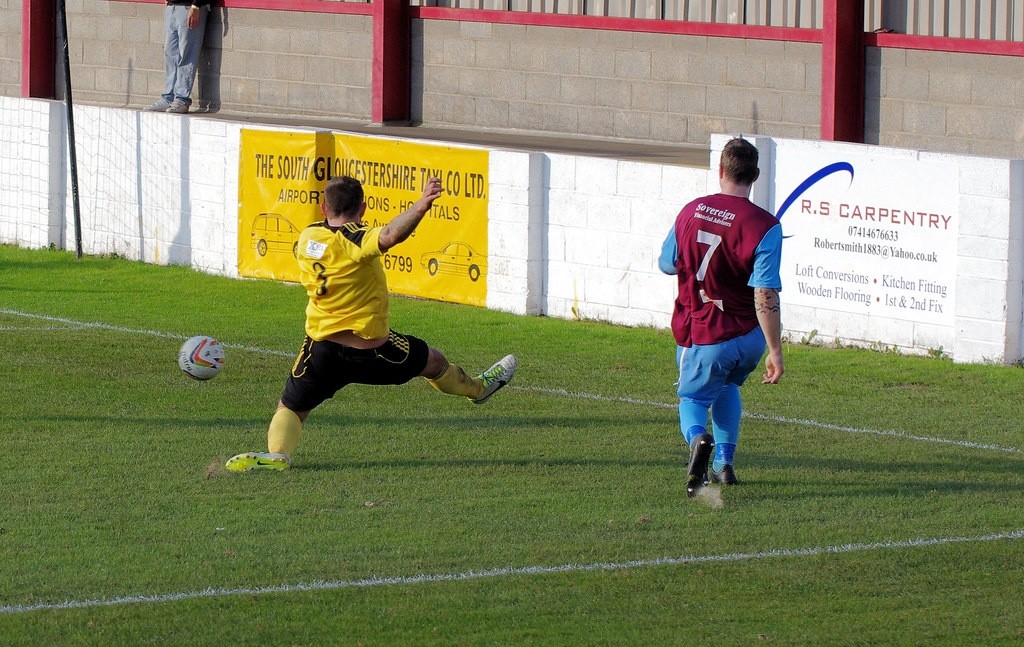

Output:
xmin=708 ymin=461 xmax=738 ymax=485
xmin=226 ymin=452 xmax=291 ymax=473
xmin=467 ymin=352 xmax=517 ymax=405
xmin=686 ymin=433 xmax=716 ymax=498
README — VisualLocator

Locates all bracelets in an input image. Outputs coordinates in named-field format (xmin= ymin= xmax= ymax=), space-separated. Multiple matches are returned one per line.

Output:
xmin=191 ymin=5 xmax=199 ymax=9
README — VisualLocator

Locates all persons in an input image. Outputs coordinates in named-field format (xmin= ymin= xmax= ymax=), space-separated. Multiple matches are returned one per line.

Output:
xmin=143 ymin=0 xmax=208 ymax=112
xmin=658 ymin=138 xmax=783 ymax=500
xmin=226 ymin=177 xmax=516 ymax=472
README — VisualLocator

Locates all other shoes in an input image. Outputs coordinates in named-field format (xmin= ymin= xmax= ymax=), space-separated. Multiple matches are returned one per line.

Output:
xmin=144 ymin=98 xmax=173 ymax=111
xmin=166 ymin=98 xmax=189 ymax=113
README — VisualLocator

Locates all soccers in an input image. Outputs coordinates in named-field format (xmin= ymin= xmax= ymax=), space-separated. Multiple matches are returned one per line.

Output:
xmin=177 ymin=335 xmax=228 ymax=382
